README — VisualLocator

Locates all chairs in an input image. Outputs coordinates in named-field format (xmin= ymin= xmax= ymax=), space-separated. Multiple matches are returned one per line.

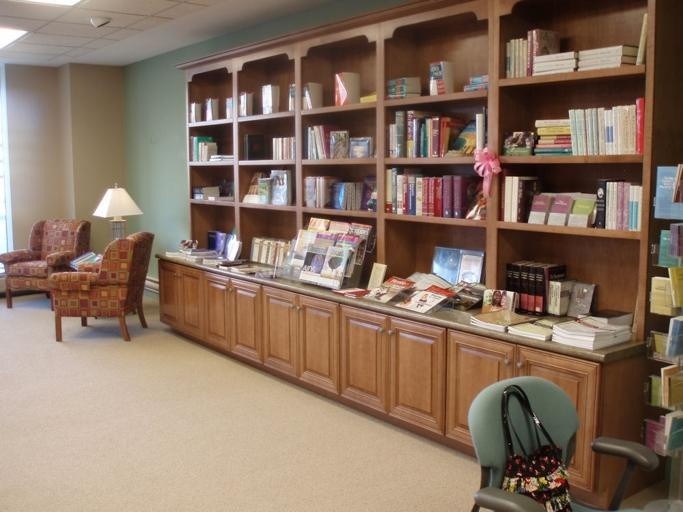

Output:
xmin=1 ymin=219 xmax=154 ymax=342
xmin=468 ymin=374 xmax=660 ymax=512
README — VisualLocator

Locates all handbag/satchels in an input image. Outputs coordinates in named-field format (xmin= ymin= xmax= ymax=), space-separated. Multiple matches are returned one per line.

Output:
xmin=501 ymin=385 xmax=573 ymax=512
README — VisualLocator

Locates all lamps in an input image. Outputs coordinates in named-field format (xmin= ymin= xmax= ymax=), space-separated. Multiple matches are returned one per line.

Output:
xmin=93 ymin=182 xmax=144 ymax=241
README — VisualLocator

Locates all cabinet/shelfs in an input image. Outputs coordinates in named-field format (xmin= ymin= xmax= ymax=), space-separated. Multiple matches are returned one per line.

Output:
xmin=155 ymin=0 xmax=680 ymax=512
